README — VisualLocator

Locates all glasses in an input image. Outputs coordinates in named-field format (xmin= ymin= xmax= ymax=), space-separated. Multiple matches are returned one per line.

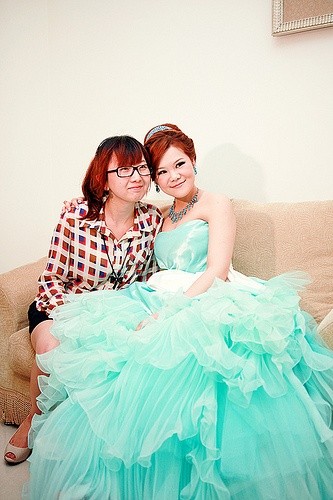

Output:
xmin=107 ymin=164 xmax=151 ymax=178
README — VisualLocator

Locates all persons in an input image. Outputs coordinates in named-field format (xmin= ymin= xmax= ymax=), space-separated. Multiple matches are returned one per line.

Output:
xmin=4 ymin=135 xmax=162 ymax=462
xmin=23 ymin=124 xmax=333 ymax=500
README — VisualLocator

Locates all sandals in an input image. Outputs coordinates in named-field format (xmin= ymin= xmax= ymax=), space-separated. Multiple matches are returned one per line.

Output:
xmin=3 ymin=419 xmax=32 ymax=463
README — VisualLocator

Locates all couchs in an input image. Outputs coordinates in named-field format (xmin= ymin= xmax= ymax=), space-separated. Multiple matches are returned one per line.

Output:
xmin=0 ymin=201 xmax=333 ymax=426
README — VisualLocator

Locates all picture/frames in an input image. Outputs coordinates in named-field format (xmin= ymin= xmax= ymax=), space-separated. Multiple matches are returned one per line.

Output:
xmin=271 ymin=0 xmax=333 ymax=37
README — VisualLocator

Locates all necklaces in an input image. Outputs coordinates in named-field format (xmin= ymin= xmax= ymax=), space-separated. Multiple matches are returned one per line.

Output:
xmin=167 ymin=188 xmax=199 ymax=224
xmin=102 ymin=237 xmax=132 ymax=289
